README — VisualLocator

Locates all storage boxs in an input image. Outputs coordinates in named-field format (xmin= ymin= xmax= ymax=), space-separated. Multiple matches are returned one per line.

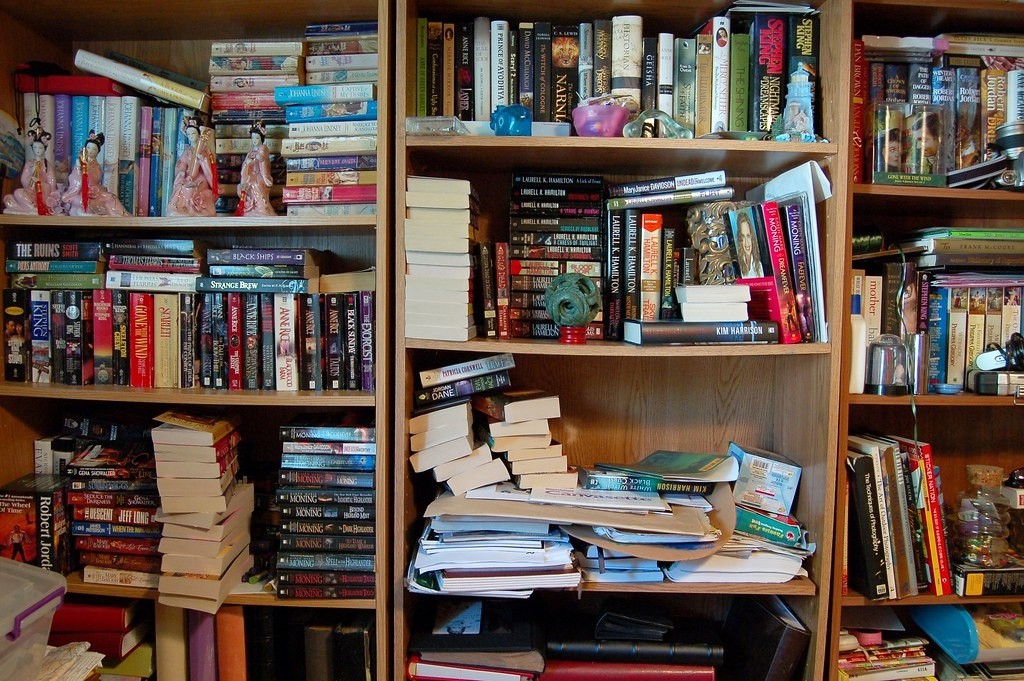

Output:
xmin=952 ymin=560 xmax=1022 ymax=599
xmin=0 ymin=556 xmax=67 ymax=681
xmin=726 ymin=441 xmax=803 ymax=517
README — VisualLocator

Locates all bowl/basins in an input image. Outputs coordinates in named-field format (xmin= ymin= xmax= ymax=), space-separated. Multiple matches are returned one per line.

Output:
xmin=571 ymin=104 xmax=630 ymax=136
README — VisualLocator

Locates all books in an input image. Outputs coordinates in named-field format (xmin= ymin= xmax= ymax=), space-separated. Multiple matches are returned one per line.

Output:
xmin=404 ymin=160 xmax=833 ymax=346
xmin=3 ymin=232 xmax=376 ymax=392
xmin=853 ymin=30 xmax=1024 ymax=191
xmin=416 ymin=0 xmax=823 ymax=141
xmin=406 ymin=598 xmax=812 ymax=681
xmin=851 ymin=225 xmax=1024 ymax=392
xmin=0 ymin=407 xmax=376 ymax=614
xmin=405 ymin=353 xmax=816 ymax=599
xmin=13 ymin=22 xmax=377 ymax=216
xmin=47 ymin=605 xmax=376 ymax=681
xmin=837 ymin=606 xmax=1024 ymax=681
xmin=842 ymin=433 xmax=955 ymax=602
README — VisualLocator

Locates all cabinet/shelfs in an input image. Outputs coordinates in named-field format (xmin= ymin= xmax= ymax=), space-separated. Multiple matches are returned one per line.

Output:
xmin=0 ymin=0 xmax=392 ymax=681
xmin=384 ymin=1 xmax=851 ymax=681
xmin=825 ymin=1 xmax=1023 ymax=681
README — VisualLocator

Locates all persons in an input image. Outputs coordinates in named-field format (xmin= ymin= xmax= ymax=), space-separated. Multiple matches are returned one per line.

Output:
xmin=912 ymin=111 xmax=943 ymax=174
xmin=874 ymin=127 xmax=905 ymax=173
xmin=736 ymin=212 xmax=764 ymax=278
xmin=234 ymin=121 xmax=278 ymax=217
xmin=3 ymin=126 xmax=67 ymax=215
xmin=61 ymin=129 xmax=134 ymax=217
xmin=166 ymin=116 xmax=224 ymax=217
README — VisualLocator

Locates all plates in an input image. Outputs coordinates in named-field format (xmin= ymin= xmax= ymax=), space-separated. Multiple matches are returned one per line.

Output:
xmin=718 ymin=131 xmax=768 ymax=140
xmin=932 ymin=383 xmax=963 ymax=395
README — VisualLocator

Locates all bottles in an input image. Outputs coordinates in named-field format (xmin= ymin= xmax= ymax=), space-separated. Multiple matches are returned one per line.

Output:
xmin=849 ymin=294 xmax=867 ymax=395
